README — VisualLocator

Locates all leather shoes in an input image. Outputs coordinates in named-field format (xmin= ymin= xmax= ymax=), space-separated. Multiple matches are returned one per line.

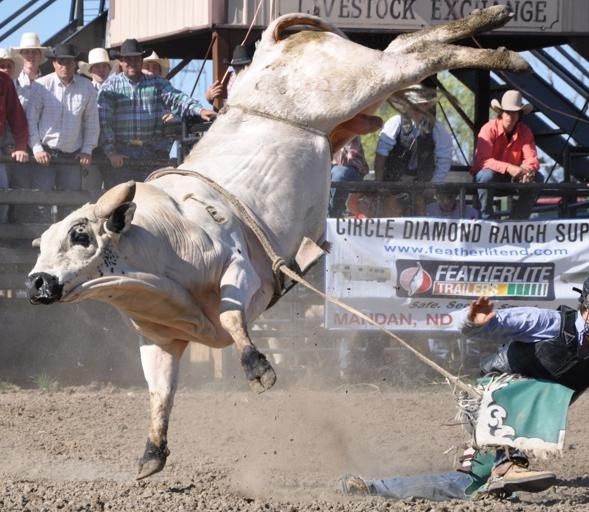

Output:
xmin=486 ymin=462 xmax=556 ymax=498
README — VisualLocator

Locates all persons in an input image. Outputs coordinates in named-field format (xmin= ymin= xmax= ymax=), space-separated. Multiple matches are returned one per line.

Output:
xmin=0 ymin=31 xmax=250 ymax=223
xmin=330 ymin=83 xmax=543 ymax=222
xmin=338 ymin=277 xmax=589 ymax=502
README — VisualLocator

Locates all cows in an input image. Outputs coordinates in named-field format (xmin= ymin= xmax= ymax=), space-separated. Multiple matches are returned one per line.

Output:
xmin=26 ymin=5 xmax=531 ymax=482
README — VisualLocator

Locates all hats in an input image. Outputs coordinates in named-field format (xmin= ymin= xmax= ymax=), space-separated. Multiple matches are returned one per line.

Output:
xmin=139 ymin=48 xmax=170 ymax=78
xmin=398 ymin=84 xmax=438 ymax=109
xmin=11 ymin=32 xmax=49 ymax=52
xmin=573 ymin=277 xmax=589 ymax=308
xmin=43 ymin=43 xmax=82 ymax=59
xmin=77 ymin=47 xmax=116 ymax=79
xmin=221 ymin=45 xmax=254 ymax=66
xmin=109 ymin=38 xmax=147 ymax=57
xmin=0 ymin=48 xmax=26 ymax=79
xmin=487 ymin=88 xmax=535 ymax=114
